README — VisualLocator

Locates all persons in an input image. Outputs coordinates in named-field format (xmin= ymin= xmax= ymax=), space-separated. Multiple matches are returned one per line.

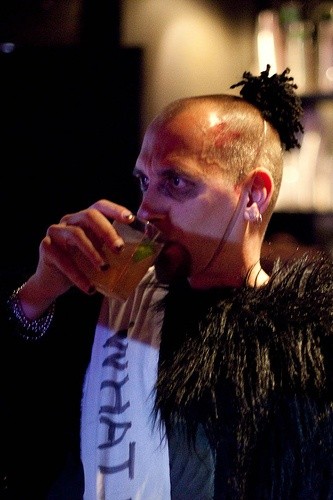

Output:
xmin=0 ymin=65 xmax=333 ymax=500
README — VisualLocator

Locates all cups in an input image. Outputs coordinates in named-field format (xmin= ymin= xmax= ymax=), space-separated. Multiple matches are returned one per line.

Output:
xmin=92 ymin=214 xmax=166 ymax=301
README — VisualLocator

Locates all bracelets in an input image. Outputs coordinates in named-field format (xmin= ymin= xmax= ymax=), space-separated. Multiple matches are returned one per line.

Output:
xmin=7 ymin=281 xmax=56 ymax=341
